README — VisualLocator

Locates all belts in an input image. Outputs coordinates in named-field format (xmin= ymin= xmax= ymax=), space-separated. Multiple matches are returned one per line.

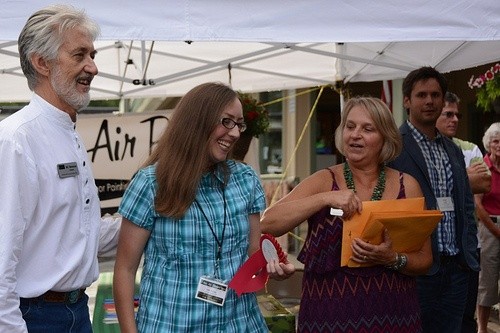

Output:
xmin=20 ymin=289 xmax=86 ymax=304
xmin=489 ymin=216 xmax=499 ymax=224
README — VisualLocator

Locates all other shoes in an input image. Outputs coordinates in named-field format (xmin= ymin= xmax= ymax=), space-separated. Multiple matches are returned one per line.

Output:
xmin=477 ymin=323 xmax=486 ymax=333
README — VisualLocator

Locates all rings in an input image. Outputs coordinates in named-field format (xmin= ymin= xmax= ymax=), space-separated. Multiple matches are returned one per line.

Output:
xmin=363 ymin=256 xmax=366 ymax=260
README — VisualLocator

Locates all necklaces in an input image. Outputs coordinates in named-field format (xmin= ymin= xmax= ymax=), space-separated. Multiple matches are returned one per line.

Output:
xmin=341 ymin=161 xmax=386 ymax=202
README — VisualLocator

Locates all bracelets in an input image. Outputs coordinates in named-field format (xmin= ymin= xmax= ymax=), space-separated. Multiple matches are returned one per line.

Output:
xmin=385 ymin=252 xmax=408 ymax=272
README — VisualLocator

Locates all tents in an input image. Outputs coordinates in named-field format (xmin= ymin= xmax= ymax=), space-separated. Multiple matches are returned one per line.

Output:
xmin=0 ymin=0 xmax=500 ymax=121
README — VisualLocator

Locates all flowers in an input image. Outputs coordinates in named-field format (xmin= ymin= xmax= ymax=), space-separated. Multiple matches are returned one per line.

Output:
xmin=237 ymin=90 xmax=272 ymax=139
xmin=467 ymin=63 xmax=500 ymax=112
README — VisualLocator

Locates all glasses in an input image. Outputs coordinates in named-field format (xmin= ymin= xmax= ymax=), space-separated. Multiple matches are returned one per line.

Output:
xmin=220 ymin=117 xmax=248 ymax=132
xmin=441 ymin=111 xmax=460 ymax=119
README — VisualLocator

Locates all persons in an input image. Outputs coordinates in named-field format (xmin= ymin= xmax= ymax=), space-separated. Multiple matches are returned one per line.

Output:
xmin=386 ymin=66 xmax=481 ymax=333
xmin=113 ymin=82 xmax=295 ymax=333
xmin=260 ymin=98 xmax=433 ymax=333
xmin=0 ymin=4 xmax=123 ymax=333
xmin=436 ymin=89 xmax=491 ymax=333
xmin=472 ymin=121 xmax=500 ymax=333
